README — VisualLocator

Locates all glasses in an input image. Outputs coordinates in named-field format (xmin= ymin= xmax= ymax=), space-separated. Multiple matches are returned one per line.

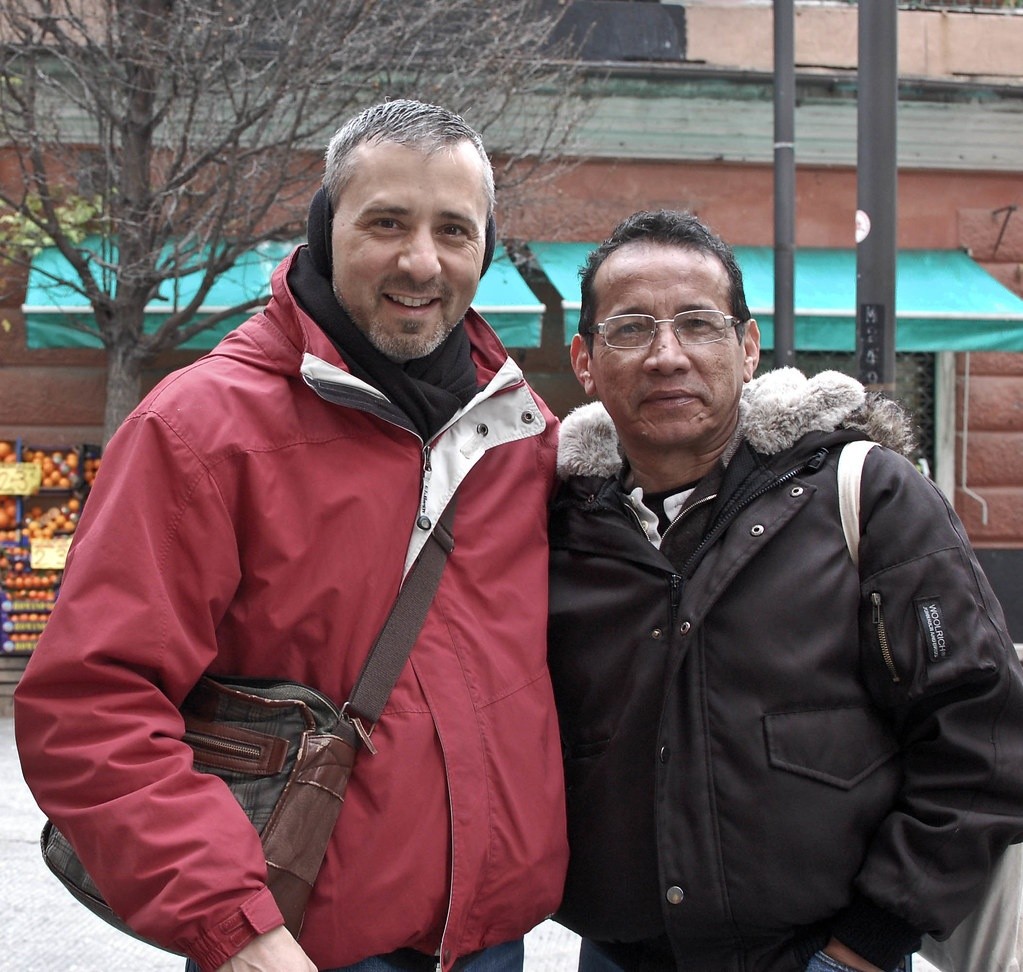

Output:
xmin=588 ymin=309 xmax=742 ymax=349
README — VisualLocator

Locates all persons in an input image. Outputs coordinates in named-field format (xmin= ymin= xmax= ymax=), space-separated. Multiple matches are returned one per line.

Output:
xmin=534 ymin=207 xmax=1022 ymax=971
xmin=13 ymin=100 xmax=578 ymax=972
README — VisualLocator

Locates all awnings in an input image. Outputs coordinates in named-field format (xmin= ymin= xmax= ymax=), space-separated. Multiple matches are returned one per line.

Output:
xmin=24 ymin=230 xmax=1023 ymax=365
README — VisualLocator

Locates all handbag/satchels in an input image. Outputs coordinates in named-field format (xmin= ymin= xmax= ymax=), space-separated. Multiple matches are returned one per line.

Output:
xmin=42 ymin=482 xmax=461 ymax=962
xmin=837 ymin=438 xmax=1022 ymax=971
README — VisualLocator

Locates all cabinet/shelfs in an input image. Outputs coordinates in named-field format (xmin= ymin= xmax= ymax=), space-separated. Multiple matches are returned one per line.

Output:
xmin=0 ymin=435 xmax=106 ymax=656
